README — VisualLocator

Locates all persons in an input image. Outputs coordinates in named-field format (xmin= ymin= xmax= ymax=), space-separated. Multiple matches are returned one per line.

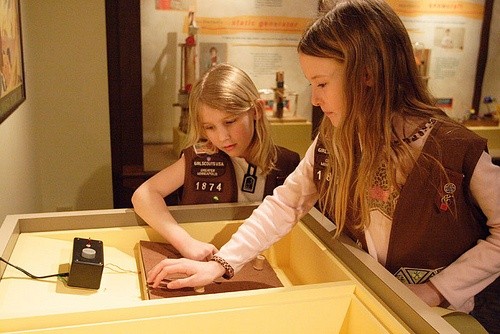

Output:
xmin=147 ymin=1 xmax=500 ymax=334
xmin=131 ymin=62 xmax=301 ymax=262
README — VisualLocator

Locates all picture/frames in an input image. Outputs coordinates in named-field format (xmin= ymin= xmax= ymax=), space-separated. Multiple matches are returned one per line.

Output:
xmin=0 ymin=0 xmax=27 ymax=124
